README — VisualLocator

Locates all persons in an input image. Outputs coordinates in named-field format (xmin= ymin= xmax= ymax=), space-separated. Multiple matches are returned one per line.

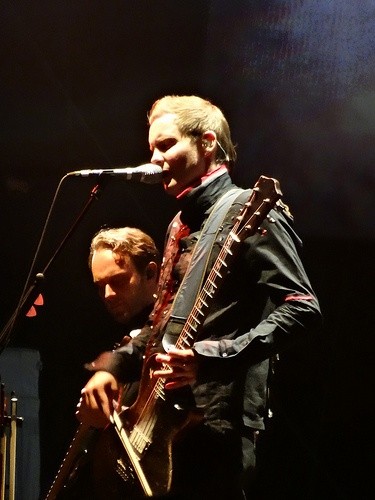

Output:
xmin=88 ymin=226 xmax=161 ymax=360
xmin=81 ymin=95 xmax=324 ymax=500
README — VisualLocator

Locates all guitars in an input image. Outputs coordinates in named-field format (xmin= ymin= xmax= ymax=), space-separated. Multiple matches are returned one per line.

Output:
xmin=46 ymin=328 xmax=141 ymax=500
xmin=94 ymin=175 xmax=293 ymax=500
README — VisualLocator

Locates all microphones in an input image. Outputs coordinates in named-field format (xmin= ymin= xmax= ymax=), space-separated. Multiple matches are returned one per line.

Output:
xmin=69 ymin=163 xmax=163 ymax=185
